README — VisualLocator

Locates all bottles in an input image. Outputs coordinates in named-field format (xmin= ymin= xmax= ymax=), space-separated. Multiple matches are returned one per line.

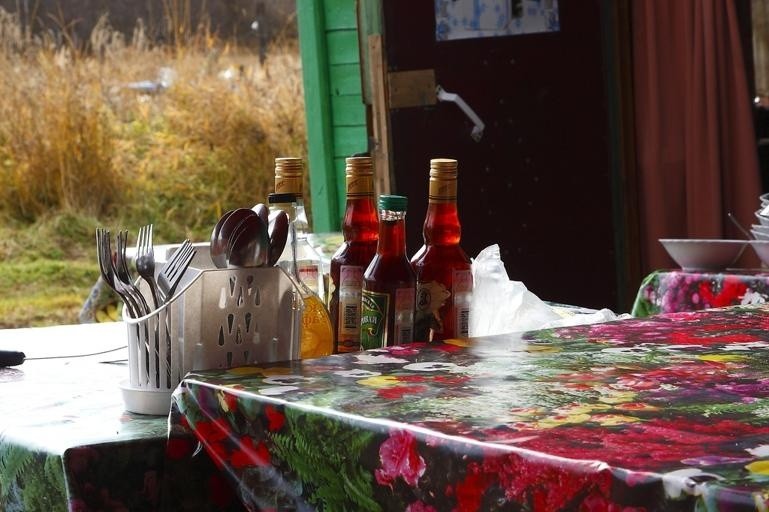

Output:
xmin=409 ymin=157 xmax=473 ymax=342
xmin=274 ymin=156 xmax=327 ymax=304
xmin=359 ymin=194 xmax=416 ymax=351
xmin=266 ymin=190 xmax=335 ymax=359
xmin=326 ymin=157 xmax=379 ymax=354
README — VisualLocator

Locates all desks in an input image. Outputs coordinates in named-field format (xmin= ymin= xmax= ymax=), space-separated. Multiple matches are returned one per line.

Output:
xmin=4 ymin=242 xmax=769 ymax=512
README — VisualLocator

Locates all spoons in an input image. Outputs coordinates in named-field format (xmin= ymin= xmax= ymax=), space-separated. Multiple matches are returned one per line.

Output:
xmin=207 ymin=203 xmax=289 ymax=285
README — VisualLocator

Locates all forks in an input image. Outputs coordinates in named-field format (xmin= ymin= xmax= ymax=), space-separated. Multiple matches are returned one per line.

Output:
xmin=94 ymin=222 xmax=198 ymax=390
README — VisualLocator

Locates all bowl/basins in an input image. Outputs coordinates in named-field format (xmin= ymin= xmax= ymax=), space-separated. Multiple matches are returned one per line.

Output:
xmin=657 ymin=191 xmax=769 ymax=272
